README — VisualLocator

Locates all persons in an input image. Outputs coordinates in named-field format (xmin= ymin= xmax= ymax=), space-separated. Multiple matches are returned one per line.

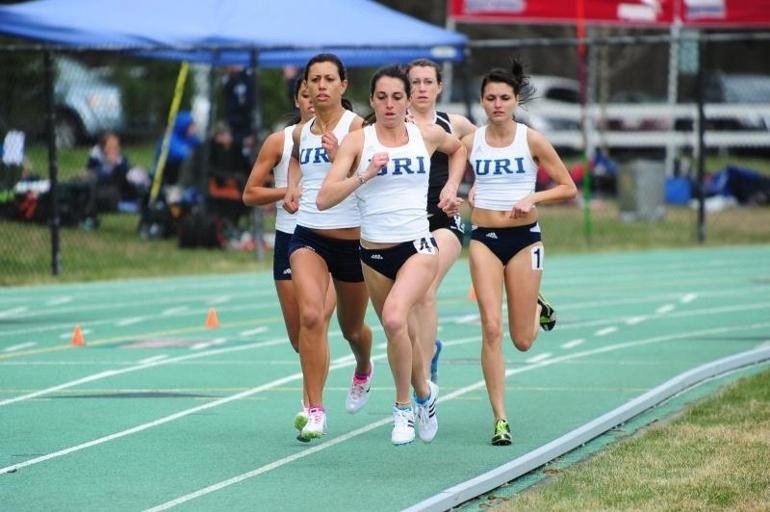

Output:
xmin=241 ymin=73 xmax=337 ymax=443
xmin=154 ymin=110 xmax=202 ymax=186
xmin=314 ymin=65 xmax=467 ymax=446
xmin=86 ymin=131 xmax=141 ymax=213
xmin=282 ymin=54 xmax=375 ymax=437
xmin=188 ymin=118 xmax=252 ymax=218
xmin=405 ymin=58 xmax=479 ymax=385
xmin=461 ymin=60 xmax=579 ymax=446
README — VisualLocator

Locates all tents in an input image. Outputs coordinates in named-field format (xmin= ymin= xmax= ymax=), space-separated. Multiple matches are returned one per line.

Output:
xmin=442 ymin=0 xmax=770 ymax=244
xmin=0 ymin=0 xmax=471 ymax=275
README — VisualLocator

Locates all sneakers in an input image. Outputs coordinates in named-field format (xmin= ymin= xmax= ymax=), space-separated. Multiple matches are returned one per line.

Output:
xmin=346 ymin=358 xmax=374 ymax=414
xmin=392 ymin=403 xmax=416 ymax=445
xmin=490 ymin=418 xmax=513 ymax=447
xmin=412 ymin=379 xmax=440 ymax=444
xmin=537 ymin=293 xmax=556 ymax=333
xmin=302 ymin=403 xmax=328 ymax=441
xmin=427 ymin=341 xmax=443 ymax=386
xmin=296 ymin=406 xmax=313 ymax=445
